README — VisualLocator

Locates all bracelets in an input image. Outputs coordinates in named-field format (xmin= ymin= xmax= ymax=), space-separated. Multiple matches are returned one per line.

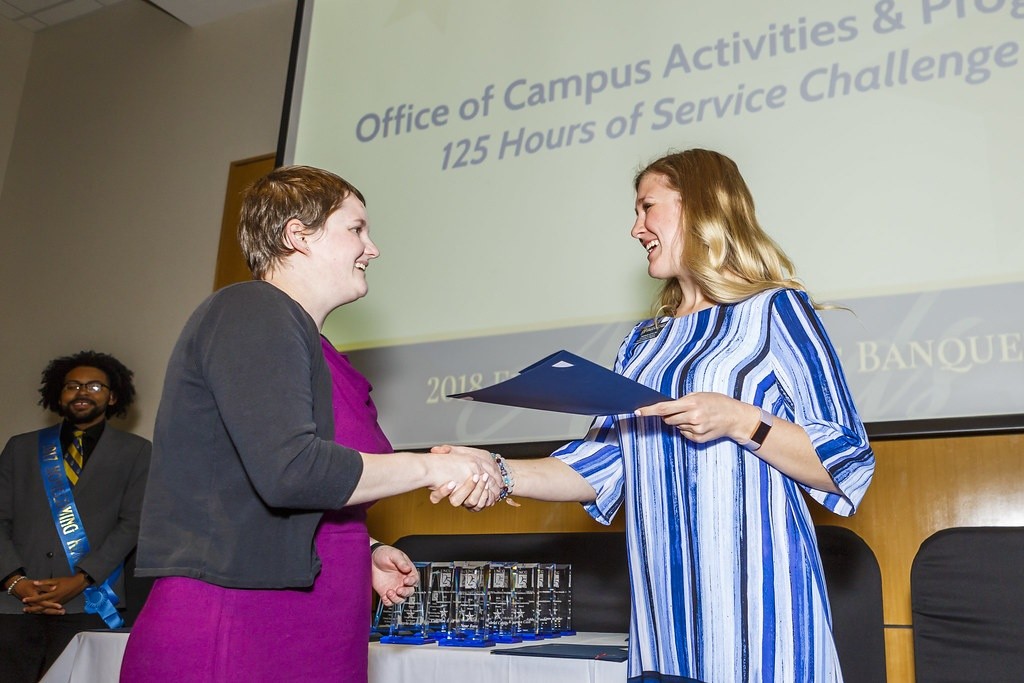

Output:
xmin=737 ymin=405 xmax=773 ymax=452
xmin=370 ymin=541 xmax=385 ymax=554
xmin=491 ymin=452 xmax=515 ymax=499
xmin=7 ymin=576 xmax=28 ymax=595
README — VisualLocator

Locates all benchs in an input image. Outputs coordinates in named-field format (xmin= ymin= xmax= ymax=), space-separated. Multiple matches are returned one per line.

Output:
xmin=376 ymin=526 xmax=887 ymax=683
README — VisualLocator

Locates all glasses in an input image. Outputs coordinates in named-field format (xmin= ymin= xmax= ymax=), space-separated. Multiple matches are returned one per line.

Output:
xmin=62 ymin=381 xmax=113 ymax=394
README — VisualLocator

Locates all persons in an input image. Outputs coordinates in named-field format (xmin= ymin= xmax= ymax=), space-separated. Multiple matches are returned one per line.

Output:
xmin=1 ymin=351 xmax=153 ymax=683
xmin=119 ymin=165 xmax=502 ymax=683
xmin=429 ymin=148 xmax=875 ymax=683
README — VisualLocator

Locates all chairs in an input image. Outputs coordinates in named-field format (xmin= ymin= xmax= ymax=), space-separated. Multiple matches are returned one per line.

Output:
xmin=909 ymin=526 xmax=1024 ymax=683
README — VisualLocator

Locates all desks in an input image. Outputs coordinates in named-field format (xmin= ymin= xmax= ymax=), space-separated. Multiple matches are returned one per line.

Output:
xmin=32 ymin=629 xmax=632 ymax=683
